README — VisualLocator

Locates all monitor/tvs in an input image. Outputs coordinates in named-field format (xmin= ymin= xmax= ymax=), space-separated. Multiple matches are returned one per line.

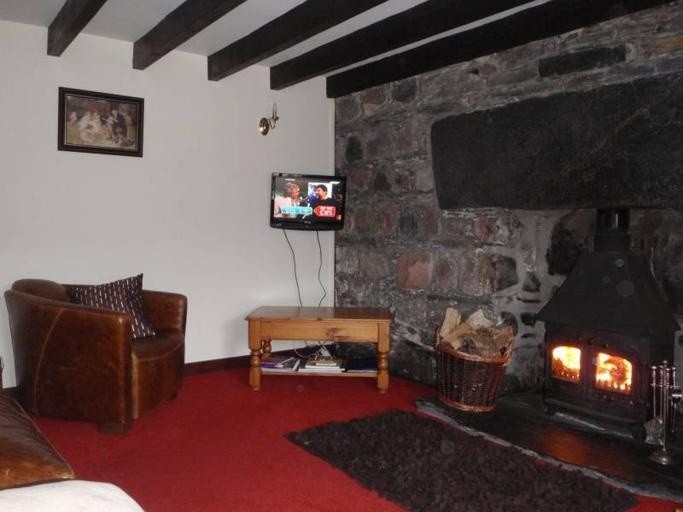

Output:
xmin=269 ymin=172 xmax=347 ymax=232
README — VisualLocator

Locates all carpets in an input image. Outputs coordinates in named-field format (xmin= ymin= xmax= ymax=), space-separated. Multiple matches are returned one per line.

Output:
xmin=281 ymin=407 xmax=637 ymax=511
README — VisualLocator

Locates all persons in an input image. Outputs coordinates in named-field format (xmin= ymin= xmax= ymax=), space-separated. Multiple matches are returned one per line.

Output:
xmin=70 ymin=109 xmax=135 ymax=148
xmin=275 ymin=182 xmax=342 ymax=224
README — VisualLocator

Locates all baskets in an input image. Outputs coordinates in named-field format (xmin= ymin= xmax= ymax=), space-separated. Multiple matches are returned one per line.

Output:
xmin=435 ymin=328 xmax=512 ymax=412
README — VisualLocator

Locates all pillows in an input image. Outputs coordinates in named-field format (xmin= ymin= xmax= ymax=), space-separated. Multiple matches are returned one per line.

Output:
xmin=62 ymin=272 xmax=159 ymax=346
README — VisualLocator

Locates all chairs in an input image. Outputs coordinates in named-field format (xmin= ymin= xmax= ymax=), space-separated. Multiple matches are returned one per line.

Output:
xmin=4 ymin=278 xmax=186 ymax=434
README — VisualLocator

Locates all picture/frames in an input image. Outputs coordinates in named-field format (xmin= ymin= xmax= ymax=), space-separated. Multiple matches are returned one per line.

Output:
xmin=57 ymin=86 xmax=144 ymax=157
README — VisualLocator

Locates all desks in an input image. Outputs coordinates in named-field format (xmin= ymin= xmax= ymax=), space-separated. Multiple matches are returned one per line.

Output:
xmin=247 ymin=305 xmax=395 ymax=394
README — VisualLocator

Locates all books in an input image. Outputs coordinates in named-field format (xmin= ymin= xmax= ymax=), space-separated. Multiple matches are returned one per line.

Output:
xmin=262 ymin=353 xmax=377 ymax=373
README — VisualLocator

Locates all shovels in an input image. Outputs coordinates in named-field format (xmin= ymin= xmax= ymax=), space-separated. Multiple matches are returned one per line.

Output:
xmin=644 ymin=366 xmax=664 ymax=446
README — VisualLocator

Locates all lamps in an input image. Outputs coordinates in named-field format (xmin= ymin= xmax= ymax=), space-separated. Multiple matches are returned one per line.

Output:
xmin=260 ymin=101 xmax=279 ymax=135
